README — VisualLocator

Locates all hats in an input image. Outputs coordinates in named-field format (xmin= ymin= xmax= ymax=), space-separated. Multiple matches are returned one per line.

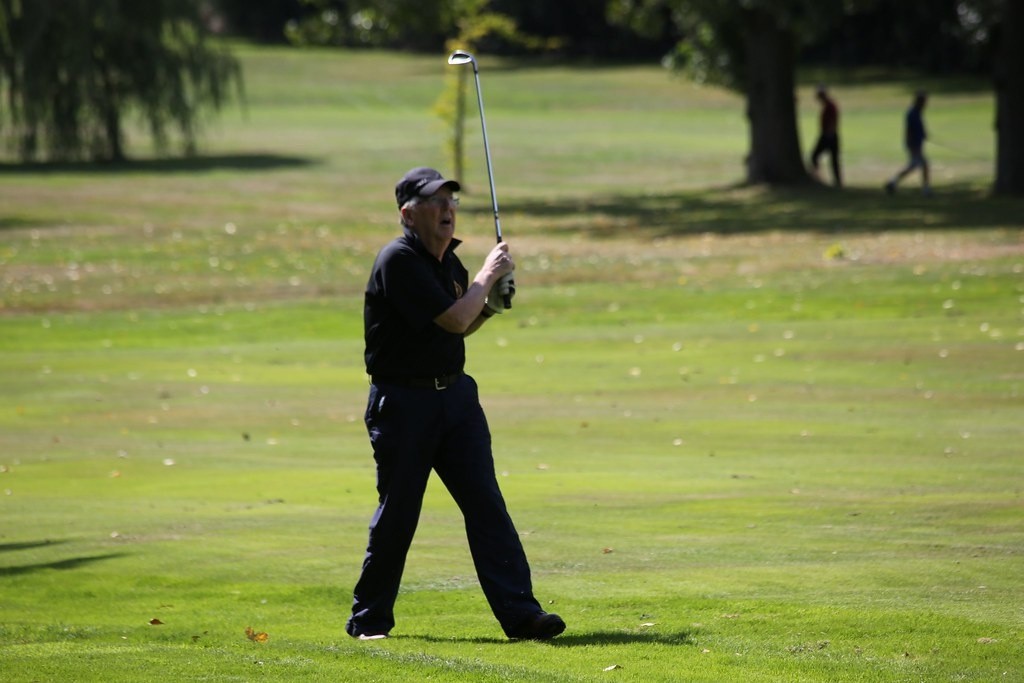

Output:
xmin=396 ymin=165 xmax=461 ymax=209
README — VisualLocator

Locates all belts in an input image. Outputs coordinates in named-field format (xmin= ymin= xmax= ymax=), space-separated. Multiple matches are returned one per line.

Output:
xmin=372 ymin=371 xmax=460 ymax=391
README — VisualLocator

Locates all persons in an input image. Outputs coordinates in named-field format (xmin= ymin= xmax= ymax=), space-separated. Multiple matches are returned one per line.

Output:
xmin=811 ymin=88 xmax=843 ymax=187
xmin=342 ymin=168 xmax=568 ymax=641
xmin=883 ymin=91 xmax=938 ymax=199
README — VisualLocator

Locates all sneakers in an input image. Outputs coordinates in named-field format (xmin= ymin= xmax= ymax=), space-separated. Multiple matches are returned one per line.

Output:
xmin=514 ymin=613 xmax=565 ymax=639
xmin=357 ymin=629 xmax=386 ymax=639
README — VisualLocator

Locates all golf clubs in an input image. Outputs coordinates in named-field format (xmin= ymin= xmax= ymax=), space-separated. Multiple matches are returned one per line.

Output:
xmin=448 ymin=50 xmax=512 ymax=309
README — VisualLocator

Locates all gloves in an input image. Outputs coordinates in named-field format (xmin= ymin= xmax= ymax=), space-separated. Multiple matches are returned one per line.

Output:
xmin=486 ymin=272 xmax=515 ymax=315
